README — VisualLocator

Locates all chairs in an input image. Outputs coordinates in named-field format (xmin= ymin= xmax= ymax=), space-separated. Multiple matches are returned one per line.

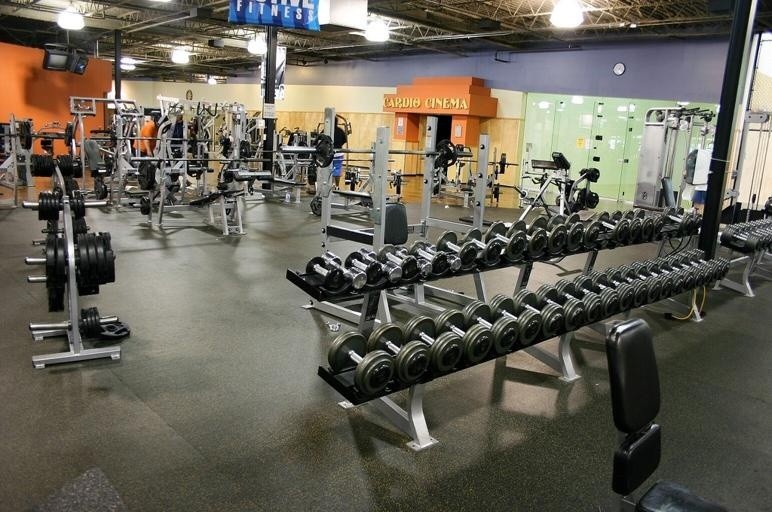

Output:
xmin=603 ymin=317 xmax=728 ymax=512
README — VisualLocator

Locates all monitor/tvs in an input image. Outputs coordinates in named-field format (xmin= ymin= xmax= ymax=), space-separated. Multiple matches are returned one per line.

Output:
xmin=71 ymin=54 xmax=89 ymax=74
xmin=42 ymin=43 xmax=71 ymax=71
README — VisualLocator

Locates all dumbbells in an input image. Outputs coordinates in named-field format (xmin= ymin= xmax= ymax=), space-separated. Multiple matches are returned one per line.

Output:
xmin=328 ymin=249 xmax=729 ymax=396
xmin=305 ymin=207 xmax=703 ymax=297
xmin=720 ymin=217 xmax=771 ymax=256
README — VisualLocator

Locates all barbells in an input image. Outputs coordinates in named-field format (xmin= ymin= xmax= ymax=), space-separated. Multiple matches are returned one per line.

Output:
xmin=280 ymin=135 xmax=473 ymax=168
xmin=89 ymin=134 xmax=276 ymax=214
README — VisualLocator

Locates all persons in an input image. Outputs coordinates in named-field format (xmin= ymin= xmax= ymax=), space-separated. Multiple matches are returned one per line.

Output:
xmin=320 ymin=116 xmax=347 ymax=190
xmin=691 ymin=184 xmax=707 ymax=216
xmin=134 ymin=114 xmax=170 ymax=190
